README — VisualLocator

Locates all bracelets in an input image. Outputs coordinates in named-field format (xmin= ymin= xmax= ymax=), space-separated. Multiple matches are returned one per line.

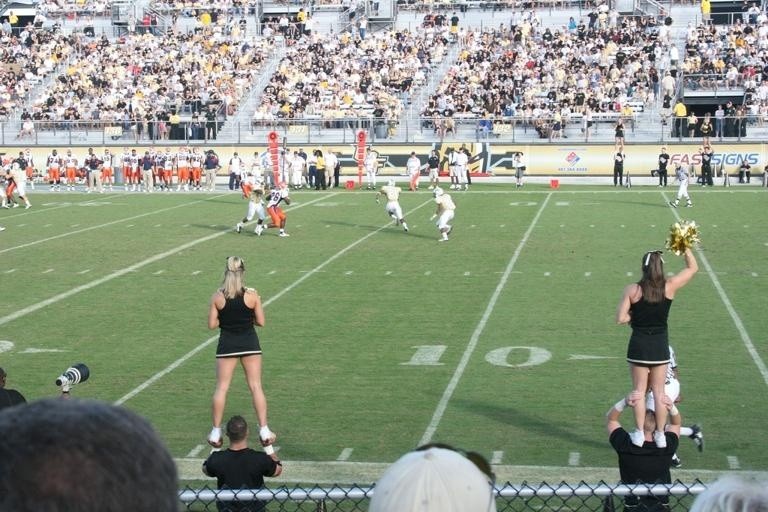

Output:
xmin=614 ymin=397 xmax=626 ymax=412
xmin=263 ymin=444 xmax=274 ymax=455
xmin=210 ymin=447 xmax=221 ymax=454
xmin=669 ymin=404 xmax=678 ymax=416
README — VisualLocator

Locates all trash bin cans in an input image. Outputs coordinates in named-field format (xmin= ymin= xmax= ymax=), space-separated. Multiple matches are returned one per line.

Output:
xmin=375 ymin=120 xmax=387 ymax=139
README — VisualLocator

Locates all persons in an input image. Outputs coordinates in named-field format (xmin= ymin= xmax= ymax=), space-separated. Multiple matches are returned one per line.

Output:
xmin=616 ymin=219 xmax=701 ymax=448
xmin=687 ymin=474 xmax=768 ymax=512
xmin=202 ymin=416 xmax=282 ymax=512
xmin=208 ymin=256 xmax=276 ymax=443
xmin=607 ymin=391 xmax=681 ymax=512
xmin=645 ymin=344 xmax=704 ymax=468
xmin=0 ymin=367 xmax=177 ymax=512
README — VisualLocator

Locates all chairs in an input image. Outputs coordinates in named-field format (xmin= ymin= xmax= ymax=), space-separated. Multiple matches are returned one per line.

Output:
xmin=2 ymin=0 xmax=276 ymax=129
xmin=421 ymin=27 xmax=668 ymax=125
xmin=680 ymin=26 xmax=768 ymax=121
xmin=252 ymin=30 xmax=460 ymax=130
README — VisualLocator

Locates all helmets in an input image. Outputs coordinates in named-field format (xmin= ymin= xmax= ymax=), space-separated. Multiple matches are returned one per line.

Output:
xmin=433 ymin=188 xmax=443 ymax=197
xmin=278 ymin=182 xmax=286 ymax=190
xmin=386 ymin=179 xmax=395 ymax=187
xmin=255 ymin=176 xmax=265 ymax=185
xmin=2 ymin=159 xmax=20 ymax=170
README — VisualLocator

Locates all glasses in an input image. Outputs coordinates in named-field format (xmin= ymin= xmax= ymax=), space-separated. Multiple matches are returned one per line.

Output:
xmin=416 ymin=443 xmax=495 ymax=512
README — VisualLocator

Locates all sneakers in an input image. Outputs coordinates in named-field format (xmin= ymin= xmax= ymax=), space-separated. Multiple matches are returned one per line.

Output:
xmin=0 ymin=203 xmax=32 ymax=209
xmin=629 ymin=431 xmax=645 ymax=448
xmin=672 ymin=457 xmax=682 ymax=467
xmin=684 ymin=204 xmax=693 ymax=208
xmin=670 ymin=202 xmax=678 ymax=208
xmin=255 ymin=224 xmax=267 ymax=236
xmin=688 ymin=424 xmax=703 ymax=451
xmin=237 ymin=223 xmax=241 ymax=233
xmin=437 ymin=225 xmax=452 ymax=242
xmin=260 ymin=429 xmax=276 ymax=441
xmin=653 ymin=430 xmax=667 ymax=448
xmin=279 ymin=232 xmax=290 ymax=237
xmin=209 ymin=428 xmax=222 ymax=443
